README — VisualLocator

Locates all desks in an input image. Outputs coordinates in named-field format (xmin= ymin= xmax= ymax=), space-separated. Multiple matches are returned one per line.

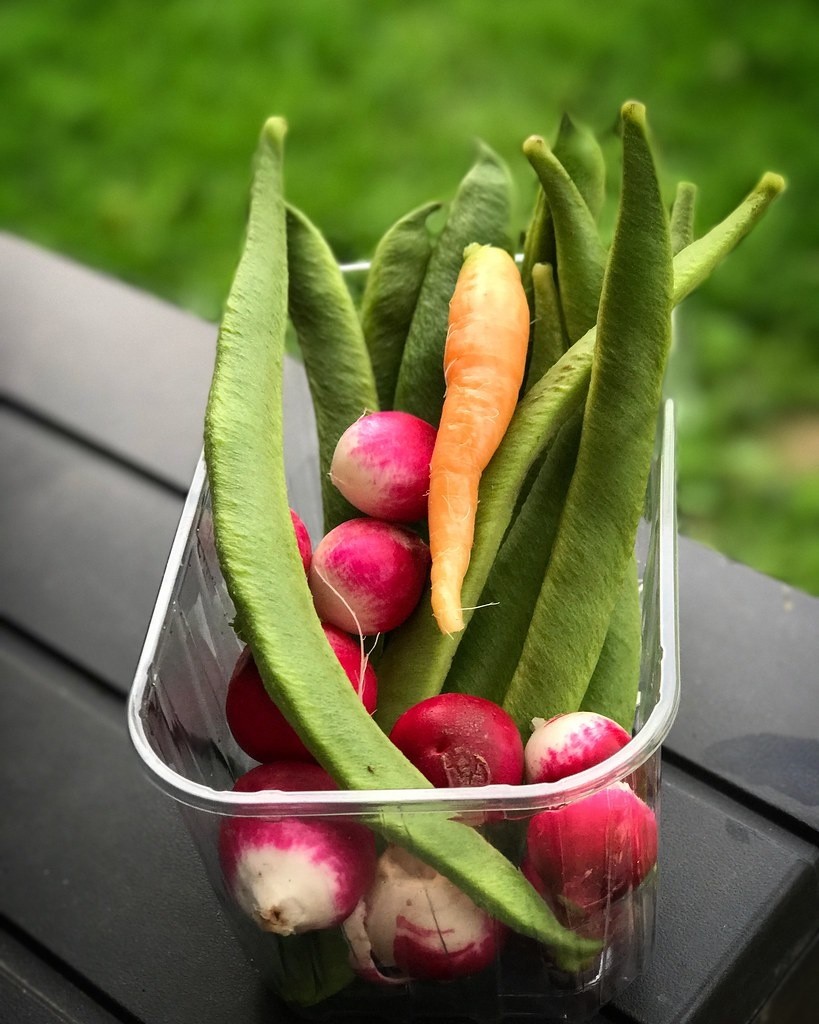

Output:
xmin=0 ymin=229 xmax=819 ymax=1024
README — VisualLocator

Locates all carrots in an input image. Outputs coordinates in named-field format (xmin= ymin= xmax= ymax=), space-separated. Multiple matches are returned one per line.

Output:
xmin=429 ymin=241 xmax=533 ymax=637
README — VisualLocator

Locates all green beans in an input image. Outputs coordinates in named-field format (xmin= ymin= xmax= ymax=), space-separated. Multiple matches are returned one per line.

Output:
xmin=205 ymin=94 xmax=787 ymax=969
